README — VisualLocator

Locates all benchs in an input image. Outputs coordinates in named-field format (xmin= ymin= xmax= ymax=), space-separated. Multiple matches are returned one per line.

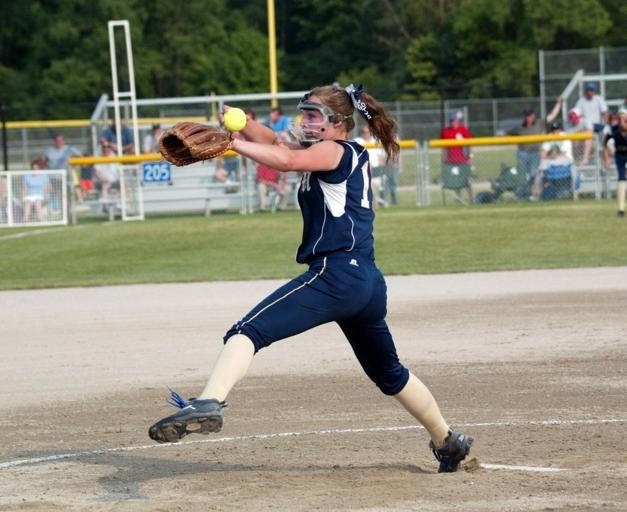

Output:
xmin=86 ymin=124 xmax=300 ymax=221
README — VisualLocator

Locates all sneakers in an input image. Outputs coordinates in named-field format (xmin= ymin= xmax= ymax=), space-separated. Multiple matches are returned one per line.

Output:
xmin=148 ymin=387 xmax=228 ymax=444
xmin=428 ymin=427 xmax=473 ymax=473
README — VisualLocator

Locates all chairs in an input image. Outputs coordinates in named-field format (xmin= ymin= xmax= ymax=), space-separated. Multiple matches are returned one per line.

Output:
xmin=440 ymin=161 xmax=473 ymax=205
xmin=369 ymin=166 xmax=390 ymax=208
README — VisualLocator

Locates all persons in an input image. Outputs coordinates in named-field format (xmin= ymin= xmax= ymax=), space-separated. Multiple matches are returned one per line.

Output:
xmin=22 ymin=117 xmax=166 ymax=222
xmin=354 ymin=125 xmax=400 ymax=207
xmin=216 ymin=107 xmax=297 ymax=213
xmin=441 ymin=111 xmax=474 ymax=203
xmin=510 ymin=81 xmax=626 ymax=215
xmin=149 ymin=82 xmax=473 ymax=472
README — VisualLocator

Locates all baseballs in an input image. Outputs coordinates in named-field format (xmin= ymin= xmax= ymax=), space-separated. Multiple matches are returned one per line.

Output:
xmin=223 ymin=108 xmax=246 ymax=131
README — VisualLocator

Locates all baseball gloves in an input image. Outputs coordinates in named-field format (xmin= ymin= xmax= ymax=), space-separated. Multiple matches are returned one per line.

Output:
xmin=159 ymin=122 xmax=231 ymax=167
